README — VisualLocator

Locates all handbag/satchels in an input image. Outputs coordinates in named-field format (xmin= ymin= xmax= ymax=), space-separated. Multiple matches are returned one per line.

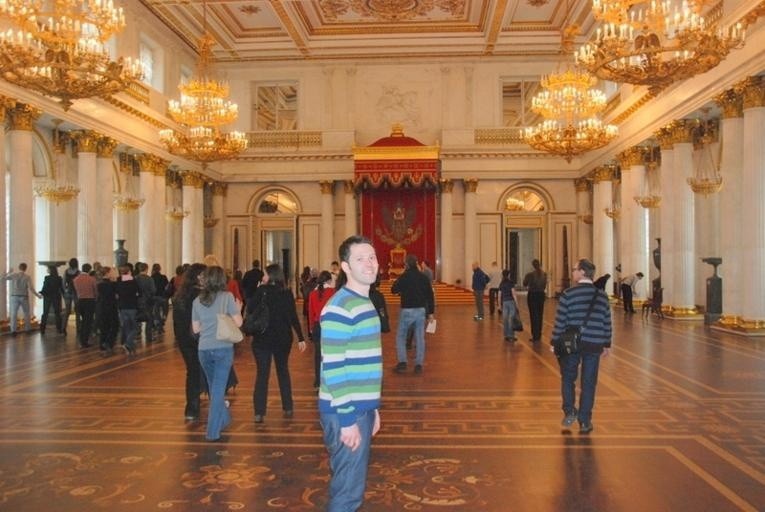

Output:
xmin=217 ymin=314 xmax=243 ymax=344
xmin=559 ymin=327 xmax=582 ymax=354
xmin=243 ymin=302 xmax=269 ymax=336
xmin=136 ymin=298 xmax=153 ymax=321
xmin=512 ymin=318 xmax=523 ymax=331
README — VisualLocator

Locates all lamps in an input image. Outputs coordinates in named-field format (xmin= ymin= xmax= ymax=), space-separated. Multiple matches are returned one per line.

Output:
xmin=204 ymin=182 xmax=220 ymax=230
xmin=574 ymin=1 xmax=746 ymax=97
xmin=112 ymin=145 xmax=146 ymax=213
xmin=1 ymin=0 xmax=147 ymax=111
xmin=603 ymin=165 xmax=623 ymax=222
xmin=686 ymin=107 xmax=726 ymax=199
xmin=159 ymin=0 xmax=250 ymax=170
xmin=165 ymin=165 xmax=190 ymax=223
xmin=577 ymin=177 xmax=593 ymax=225
xmin=634 ymin=138 xmax=662 ymax=214
xmin=518 ymin=0 xmax=619 ymax=163
xmin=34 ymin=118 xmax=80 ymax=204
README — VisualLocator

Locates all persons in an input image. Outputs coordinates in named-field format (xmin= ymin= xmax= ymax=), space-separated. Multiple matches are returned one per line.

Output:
xmin=621 ymin=272 xmax=644 ymax=313
xmin=472 ymin=260 xmax=547 ymax=344
xmin=320 ymin=236 xmax=382 ymax=512
xmin=201 ymin=255 xmax=263 ymax=396
xmin=4 ymin=263 xmax=41 ymax=336
xmin=613 ymin=265 xmax=621 ymax=297
xmin=550 ymin=257 xmax=612 ymax=432
xmin=595 ymin=274 xmax=610 ymax=291
xmin=192 ymin=265 xmax=243 ymax=441
xmin=40 ymin=258 xmax=190 ymax=356
xmin=392 ymin=253 xmax=433 ymax=373
xmin=174 ymin=263 xmax=207 ymax=427
xmin=301 ymin=260 xmax=344 ymax=385
xmin=242 ymin=264 xmax=307 ymax=423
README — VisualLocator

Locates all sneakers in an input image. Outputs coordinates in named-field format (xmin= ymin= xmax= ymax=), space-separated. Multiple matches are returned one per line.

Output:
xmin=12 ymin=322 xmax=166 ymax=357
xmin=415 ymin=365 xmax=422 ymax=374
xmin=185 ymin=385 xmax=292 ymax=442
xmin=395 ymin=361 xmax=406 ymax=373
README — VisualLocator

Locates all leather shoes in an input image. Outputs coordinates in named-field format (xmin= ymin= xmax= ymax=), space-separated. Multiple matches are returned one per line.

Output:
xmin=580 ymin=421 xmax=593 ymax=431
xmin=563 ymin=408 xmax=576 ymax=425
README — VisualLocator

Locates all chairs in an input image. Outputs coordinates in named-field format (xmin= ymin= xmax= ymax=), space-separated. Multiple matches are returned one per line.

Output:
xmin=641 ymin=288 xmax=665 ymax=320
xmin=388 ymin=245 xmax=406 ymax=280
